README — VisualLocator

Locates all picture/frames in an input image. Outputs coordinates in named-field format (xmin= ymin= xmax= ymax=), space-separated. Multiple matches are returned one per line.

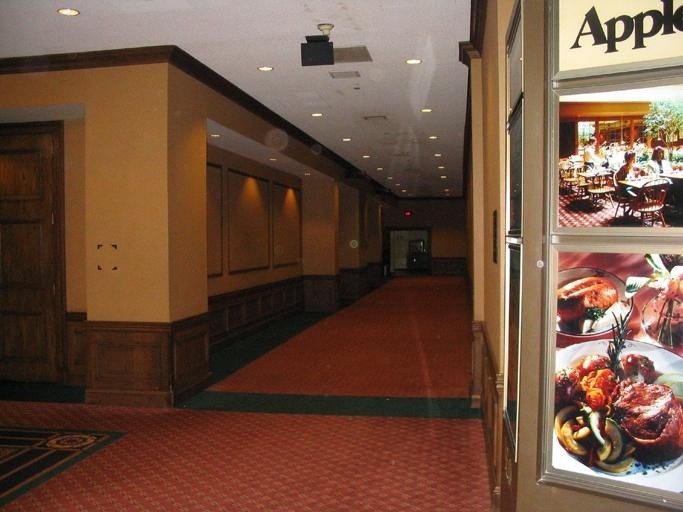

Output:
xmin=537 ymin=70 xmax=683 ymax=511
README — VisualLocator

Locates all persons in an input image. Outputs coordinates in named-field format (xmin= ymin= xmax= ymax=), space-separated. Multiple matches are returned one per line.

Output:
xmin=615 ymin=150 xmax=648 ymax=219
xmin=583 ymin=137 xmax=601 ymax=197
xmin=646 ymin=146 xmax=674 ymax=175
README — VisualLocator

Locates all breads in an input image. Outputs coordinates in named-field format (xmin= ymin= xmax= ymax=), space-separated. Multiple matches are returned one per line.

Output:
xmin=557 ymin=276 xmax=619 ymax=322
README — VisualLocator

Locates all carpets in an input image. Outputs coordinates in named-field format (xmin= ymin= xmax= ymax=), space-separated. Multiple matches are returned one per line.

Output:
xmin=1 ymin=422 xmax=127 ymax=505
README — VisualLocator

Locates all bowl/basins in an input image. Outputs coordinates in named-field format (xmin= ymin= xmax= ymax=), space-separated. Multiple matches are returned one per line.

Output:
xmin=554 ymin=265 xmax=633 ymax=344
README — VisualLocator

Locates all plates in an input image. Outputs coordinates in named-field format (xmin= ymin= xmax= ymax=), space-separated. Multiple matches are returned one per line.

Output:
xmin=555 ymin=337 xmax=682 ymax=493
